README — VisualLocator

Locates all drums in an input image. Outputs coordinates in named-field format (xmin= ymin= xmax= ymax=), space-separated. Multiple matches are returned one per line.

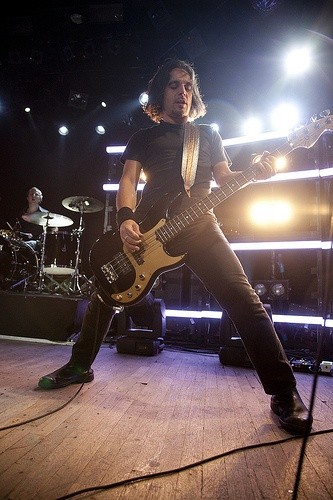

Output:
xmin=42 ymin=267 xmax=78 ymax=293
xmin=39 ymin=230 xmax=77 ymax=266
xmin=0 ymin=229 xmax=40 ymax=292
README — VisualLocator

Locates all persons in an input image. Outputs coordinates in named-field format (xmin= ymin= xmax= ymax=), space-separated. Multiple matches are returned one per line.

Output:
xmin=17 ymin=187 xmax=58 ymax=264
xmin=38 ymin=57 xmax=313 ymax=434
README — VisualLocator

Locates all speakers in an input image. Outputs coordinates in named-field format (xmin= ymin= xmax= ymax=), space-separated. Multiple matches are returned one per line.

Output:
xmin=218 ymin=303 xmax=273 ymax=370
xmin=115 ymin=300 xmax=166 ymax=355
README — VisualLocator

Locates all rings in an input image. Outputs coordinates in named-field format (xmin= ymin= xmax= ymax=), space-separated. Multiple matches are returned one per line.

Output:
xmin=124 ymin=235 xmax=128 ymax=240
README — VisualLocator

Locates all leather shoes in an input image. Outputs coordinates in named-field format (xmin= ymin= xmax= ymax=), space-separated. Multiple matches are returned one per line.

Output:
xmin=38 ymin=355 xmax=95 ymax=389
xmin=270 ymin=386 xmax=313 ymax=434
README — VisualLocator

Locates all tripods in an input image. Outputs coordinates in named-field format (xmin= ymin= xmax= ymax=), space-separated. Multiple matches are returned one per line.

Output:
xmin=9 ymin=202 xmax=98 ymax=297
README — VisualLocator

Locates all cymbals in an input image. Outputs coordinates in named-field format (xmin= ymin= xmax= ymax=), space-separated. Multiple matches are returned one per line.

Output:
xmin=61 ymin=195 xmax=101 ymax=213
xmin=21 ymin=211 xmax=74 ymax=228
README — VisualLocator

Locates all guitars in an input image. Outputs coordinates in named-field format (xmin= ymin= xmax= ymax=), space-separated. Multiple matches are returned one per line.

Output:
xmin=87 ymin=108 xmax=333 ymax=309
